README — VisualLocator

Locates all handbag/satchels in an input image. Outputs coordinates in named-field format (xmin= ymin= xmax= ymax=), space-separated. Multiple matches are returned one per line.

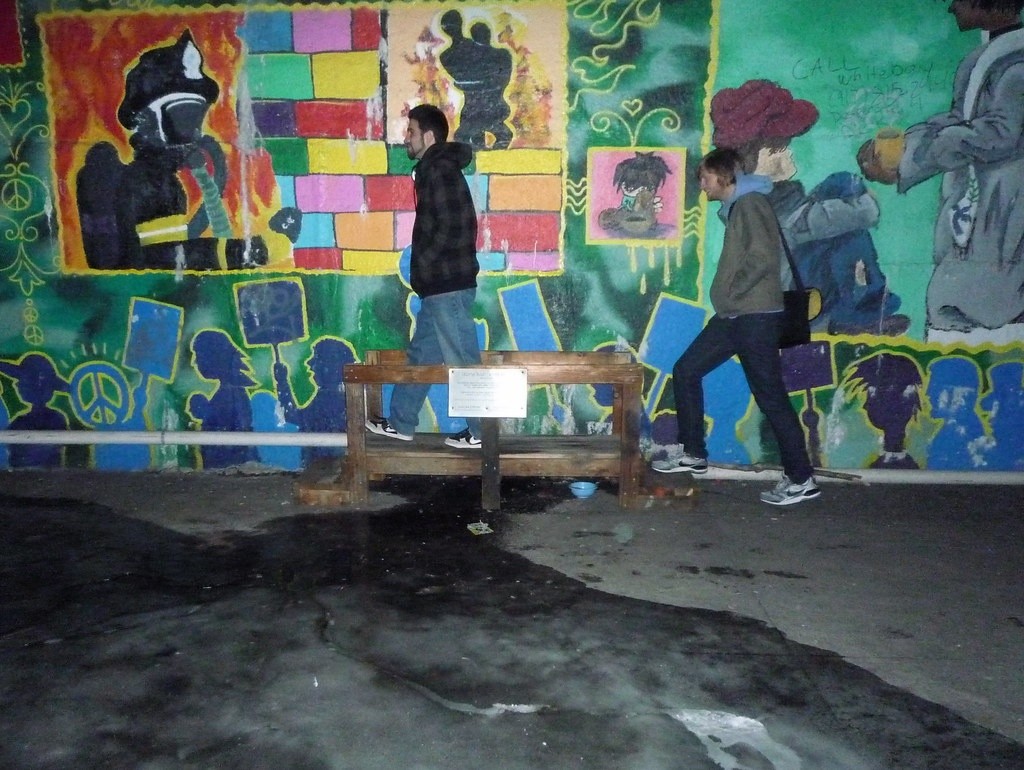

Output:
xmin=775 ymin=288 xmax=811 ymax=349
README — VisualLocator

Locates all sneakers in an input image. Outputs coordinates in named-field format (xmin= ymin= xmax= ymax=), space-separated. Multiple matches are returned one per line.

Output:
xmin=651 ymin=452 xmax=709 ymax=474
xmin=444 ymin=428 xmax=482 ymax=449
xmin=365 ymin=418 xmax=413 ymax=441
xmin=760 ymin=470 xmax=821 ymax=506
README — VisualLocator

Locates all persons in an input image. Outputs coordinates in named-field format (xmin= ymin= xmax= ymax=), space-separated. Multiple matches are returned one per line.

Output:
xmin=648 ymin=147 xmax=826 ymax=507
xmin=363 ymin=103 xmax=493 ymax=450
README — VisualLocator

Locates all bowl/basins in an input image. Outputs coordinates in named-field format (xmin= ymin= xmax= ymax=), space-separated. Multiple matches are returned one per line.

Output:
xmin=569 ymin=482 xmax=597 ymax=499
